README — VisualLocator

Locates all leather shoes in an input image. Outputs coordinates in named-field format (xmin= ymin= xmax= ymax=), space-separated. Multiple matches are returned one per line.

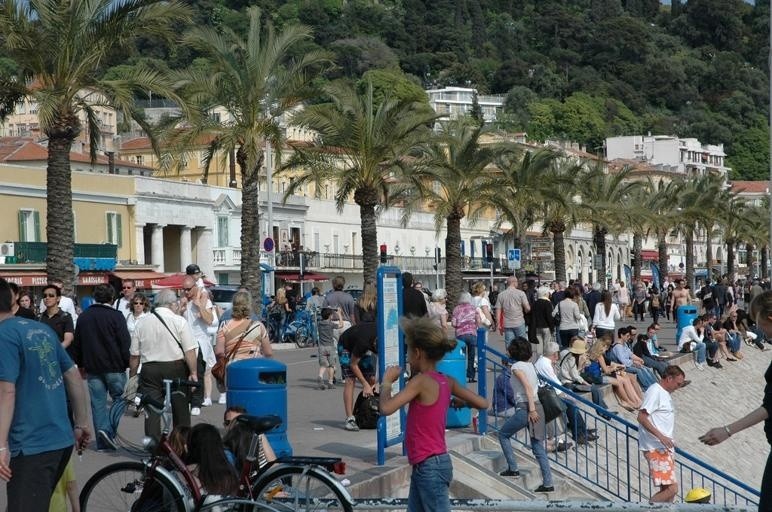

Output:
xmin=586 ymin=434 xmax=600 ymax=440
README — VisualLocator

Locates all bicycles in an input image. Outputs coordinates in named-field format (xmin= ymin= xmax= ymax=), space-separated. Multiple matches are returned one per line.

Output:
xmin=294 ymin=311 xmax=314 ymax=347
xmin=79 ymin=377 xmax=357 ymax=512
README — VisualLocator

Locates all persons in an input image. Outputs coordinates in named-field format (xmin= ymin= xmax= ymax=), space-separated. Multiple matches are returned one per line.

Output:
xmin=1 ymin=264 xmax=312 ymax=512
xmin=305 ymin=270 xmax=772 ymax=512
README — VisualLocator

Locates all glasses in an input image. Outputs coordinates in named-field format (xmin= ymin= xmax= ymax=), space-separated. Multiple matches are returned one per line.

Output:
xmin=122 ymin=287 xmax=132 ymax=290
xmin=182 ymin=284 xmax=194 ymax=291
xmin=225 ymin=420 xmax=233 ymax=425
xmin=133 ymin=302 xmax=144 ymax=306
xmin=42 ymin=294 xmax=56 ymax=299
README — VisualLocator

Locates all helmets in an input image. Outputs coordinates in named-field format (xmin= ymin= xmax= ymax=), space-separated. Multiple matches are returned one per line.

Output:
xmin=684 ymin=487 xmax=711 ymax=502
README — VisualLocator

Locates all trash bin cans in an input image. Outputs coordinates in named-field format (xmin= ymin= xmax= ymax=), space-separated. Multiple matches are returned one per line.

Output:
xmin=676 ymin=306 xmax=697 ymax=345
xmin=435 ymin=339 xmax=471 ymax=427
xmin=226 ymin=359 xmax=292 ymax=493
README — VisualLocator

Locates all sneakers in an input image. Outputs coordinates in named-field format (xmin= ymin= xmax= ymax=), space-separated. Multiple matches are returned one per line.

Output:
xmin=344 ymin=416 xmax=359 ymax=431
xmin=498 ymin=469 xmax=519 ymax=478
xmin=572 ymin=432 xmax=590 ymax=445
xmin=190 ymin=407 xmax=200 ymax=416
xmin=218 ymin=392 xmax=226 ymax=404
xmin=328 ymin=383 xmax=336 ymax=389
xmin=98 ymin=429 xmax=117 ymax=450
xmin=557 ymin=441 xmax=573 ymax=451
xmin=201 ymin=398 xmax=212 ymax=406
xmin=96 ymin=448 xmax=109 ymax=452
xmin=534 ymin=485 xmax=555 ymax=493
xmin=317 ymin=375 xmax=325 ymax=390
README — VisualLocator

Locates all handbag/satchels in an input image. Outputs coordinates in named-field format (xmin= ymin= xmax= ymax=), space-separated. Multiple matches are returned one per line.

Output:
xmin=554 ymin=312 xmax=561 ymax=325
xmin=538 ymin=385 xmax=567 ymax=423
xmin=211 ymin=357 xmax=228 ymax=382
xmin=475 ymin=307 xmax=492 ymax=330
xmin=577 ymin=373 xmax=599 ymax=384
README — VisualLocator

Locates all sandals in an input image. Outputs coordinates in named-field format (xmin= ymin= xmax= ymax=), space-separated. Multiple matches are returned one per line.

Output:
xmin=469 ymin=377 xmax=477 ymax=382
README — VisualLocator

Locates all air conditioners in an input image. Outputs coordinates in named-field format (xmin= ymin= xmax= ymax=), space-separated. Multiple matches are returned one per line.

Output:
xmin=0 ymin=243 xmax=14 ymax=257
xmin=634 ymin=144 xmax=643 ymax=152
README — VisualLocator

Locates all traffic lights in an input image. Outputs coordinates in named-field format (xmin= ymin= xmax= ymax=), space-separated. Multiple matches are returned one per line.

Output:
xmin=487 ymin=244 xmax=493 ymax=262
xmin=381 ymin=244 xmax=387 ymax=263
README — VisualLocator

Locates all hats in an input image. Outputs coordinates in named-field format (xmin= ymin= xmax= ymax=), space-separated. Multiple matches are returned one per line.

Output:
xmin=568 ymin=336 xmax=589 ymax=354
xmin=186 ymin=263 xmax=201 ymax=275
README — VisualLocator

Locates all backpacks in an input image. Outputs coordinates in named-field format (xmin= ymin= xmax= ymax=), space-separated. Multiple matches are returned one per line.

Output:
xmin=605 ymin=344 xmax=622 ymax=366
xmin=652 ymin=293 xmax=660 ymax=307
xmin=353 ymin=388 xmax=379 ymax=429
